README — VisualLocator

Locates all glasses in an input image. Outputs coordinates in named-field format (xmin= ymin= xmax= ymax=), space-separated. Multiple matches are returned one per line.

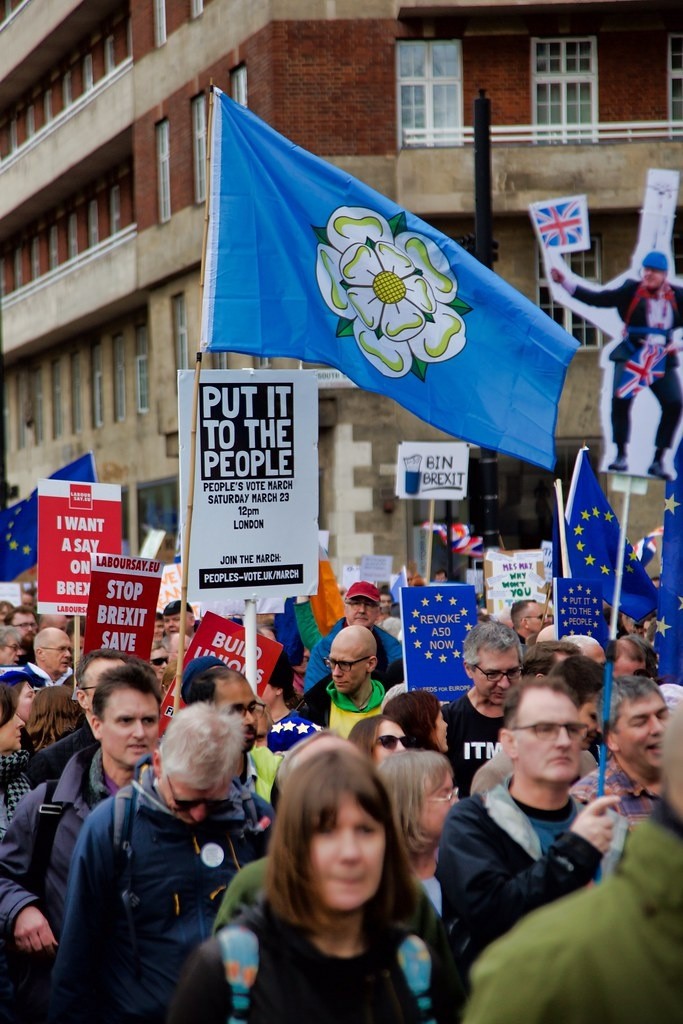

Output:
xmin=427 ymin=787 xmax=460 ymax=808
xmin=376 ymin=735 xmax=413 ymax=750
xmin=521 ymin=614 xmax=543 ymax=623
xmin=235 ymin=701 xmax=266 ymax=720
xmin=323 ymin=655 xmax=371 ymax=671
xmin=14 ymin=623 xmax=38 ymax=632
xmin=473 ymin=662 xmax=524 ymax=681
xmin=0 ymin=644 xmax=21 ymax=652
xmin=149 ymin=656 xmax=168 ymax=665
xmin=39 ymin=646 xmax=72 ymax=655
xmin=509 ymin=722 xmax=588 ymax=742
xmin=165 ymin=775 xmax=230 ymax=812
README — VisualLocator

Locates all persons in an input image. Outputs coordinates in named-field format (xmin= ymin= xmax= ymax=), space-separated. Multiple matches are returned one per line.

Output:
xmin=551 ymin=252 xmax=683 ymax=481
xmin=0 ymin=588 xmax=475 ymax=1023
xmin=306 ymin=568 xmax=683 ymax=1024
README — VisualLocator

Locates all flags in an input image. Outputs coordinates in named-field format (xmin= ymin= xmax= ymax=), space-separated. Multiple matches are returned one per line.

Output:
xmin=632 ymin=526 xmax=664 ymax=566
xmin=533 ymin=192 xmax=590 ymax=254
xmin=424 ymin=520 xmax=486 ymax=558
xmin=655 ymin=438 xmax=683 ymax=684
xmin=0 ymin=454 xmax=97 ymax=585
xmin=204 ymin=85 xmax=582 ymax=473
xmin=565 ymin=445 xmax=659 ymax=623
xmin=554 ymin=489 xmax=584 ymax=578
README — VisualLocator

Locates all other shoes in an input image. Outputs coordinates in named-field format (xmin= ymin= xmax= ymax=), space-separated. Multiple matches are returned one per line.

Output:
xmin=609 ymin=454 xmax=629 ymax=472
xmin=649 ymin=459 xmax=671 ymax=480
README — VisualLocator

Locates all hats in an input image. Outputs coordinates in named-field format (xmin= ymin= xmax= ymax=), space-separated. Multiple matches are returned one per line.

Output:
xmin=0 ymin=671 xmax=34 ymax=687
xmin=346 ymin=581 xmax=380 ymax=604
xmin=162 ymin=600 xmax=193 ymax=616
xmin=181 ymin=656 xmax=226 ymax=705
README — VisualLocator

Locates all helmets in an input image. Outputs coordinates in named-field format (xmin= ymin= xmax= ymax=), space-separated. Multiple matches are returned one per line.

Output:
xmin=643 ymin=252 xmax=669 ymax=271
